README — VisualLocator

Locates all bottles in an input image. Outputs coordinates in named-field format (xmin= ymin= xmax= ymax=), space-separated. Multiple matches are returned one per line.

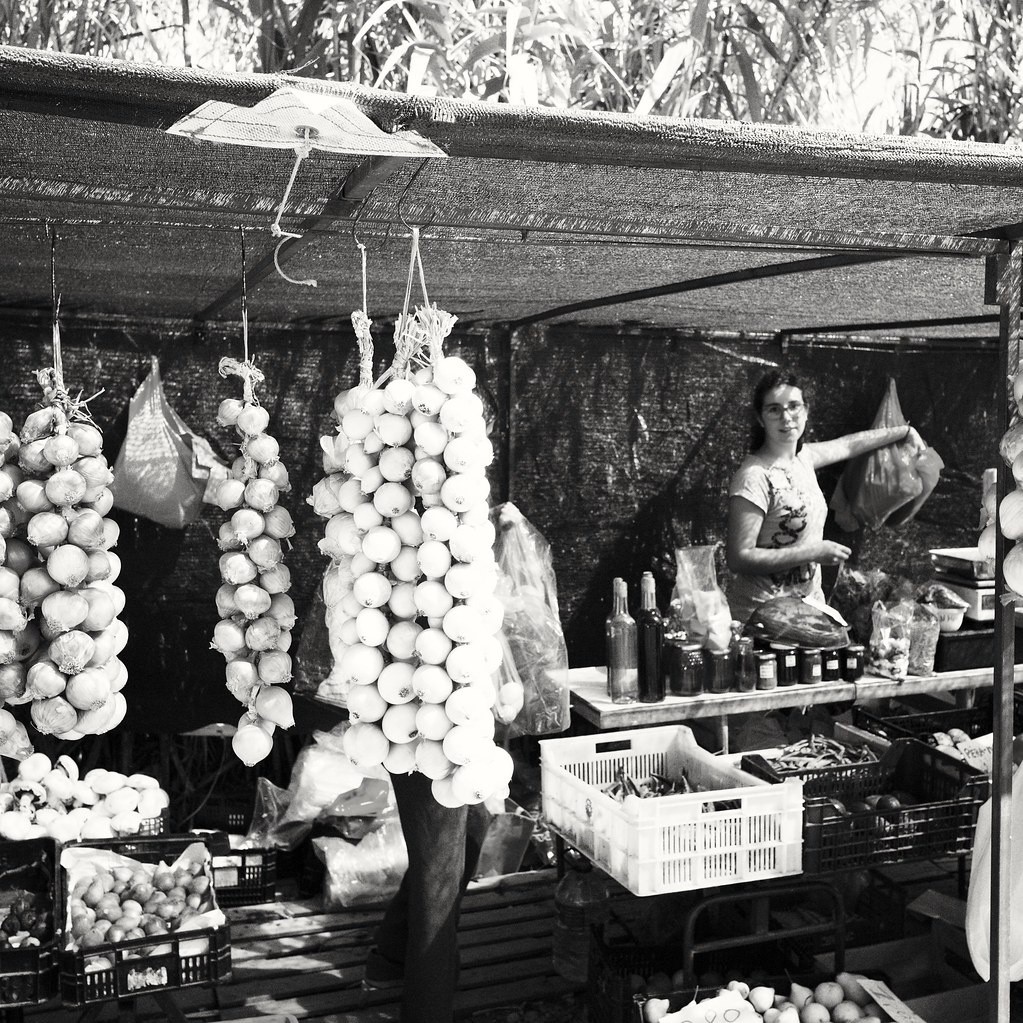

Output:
xmin=607 ymin=571 xmax=867 ymax=705
xmin=548 ymin=845 xmax=608 ymax=987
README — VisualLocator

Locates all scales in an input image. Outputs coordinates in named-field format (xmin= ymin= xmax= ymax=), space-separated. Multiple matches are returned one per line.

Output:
xmin=928 ymin=571 xmax=1023 ymax=622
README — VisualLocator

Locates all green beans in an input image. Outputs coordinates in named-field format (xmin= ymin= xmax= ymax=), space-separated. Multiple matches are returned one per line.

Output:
xmin=770 ymin=732 xmax=879 ymax=782
xmin=600 ymin=764 xmax=743 ymax=813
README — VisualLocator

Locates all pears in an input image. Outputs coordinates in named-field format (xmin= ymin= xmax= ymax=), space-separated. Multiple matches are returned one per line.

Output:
xmin=643 ymin=972 xmax=895 ymax=1022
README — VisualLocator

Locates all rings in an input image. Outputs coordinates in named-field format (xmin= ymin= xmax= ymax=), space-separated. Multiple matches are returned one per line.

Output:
xmin=832 ymin=557 xmax=839 ymax=563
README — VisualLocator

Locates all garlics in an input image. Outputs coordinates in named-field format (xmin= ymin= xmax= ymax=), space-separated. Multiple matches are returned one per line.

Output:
xmin=213 ymin=394 xmax=297 ymax=768
xmin=1000 ymin=360 xmax=1023 ymax=618
xmin=0 ymin=400 xmax=132 ymax=764
xmin=306 ymin=356 xmax=522 ymax=806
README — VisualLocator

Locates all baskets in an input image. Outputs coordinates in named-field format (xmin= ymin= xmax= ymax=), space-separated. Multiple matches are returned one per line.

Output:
xmin=214 ymin=840 xmax=273 ymax=904
xmin=127 ymin=808 xmax=174 ymax=837
xmin=56 ymin=838 xmax=231 ymax=999
xmin=881 ymin=691 xmax=1023 ymax=742
xmin=534 ymin=724 xmax=801 ymax=893
xmin=1 ymin=834 xmax=55 ymax=1006
xmin=740 ymin=735 xmax=986 ymax=863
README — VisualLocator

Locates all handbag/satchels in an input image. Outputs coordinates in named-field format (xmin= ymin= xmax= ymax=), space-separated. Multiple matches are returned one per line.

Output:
xmin=109 ymin=357 xmax=219 ymax=528
xmin=843 ymin=377 xmax=942 ymax=537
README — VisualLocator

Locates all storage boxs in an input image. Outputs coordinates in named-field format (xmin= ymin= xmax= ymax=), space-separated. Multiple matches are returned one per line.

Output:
xmin=808 ymin=929 xmax=992 ymax=1023
xmin=0 ymin=837 xmax=59 ymax=1013
xmin=739 ymin=737 xmax=990 ymax=875
xmin=632 ymin=966 xmax=895 ymax=1023
xmin=58 ymin=836 xmax=234 ymax=1010
xmin=855 ymin=689 xmax=1023 ymax=798
xmin=538 ymin=724 xmax=804 ymax=897
xmin=120 ymin=800 xmax=305 ymax=912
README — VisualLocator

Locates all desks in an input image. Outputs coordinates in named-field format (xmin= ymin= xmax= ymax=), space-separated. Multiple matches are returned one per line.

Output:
xmin=539 ymin=663 xmax=1022 ymax=754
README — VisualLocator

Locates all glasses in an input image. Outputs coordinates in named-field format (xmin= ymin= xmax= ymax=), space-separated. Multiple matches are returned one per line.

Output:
xmin=755 ymin=402 xmax=808 ymax=421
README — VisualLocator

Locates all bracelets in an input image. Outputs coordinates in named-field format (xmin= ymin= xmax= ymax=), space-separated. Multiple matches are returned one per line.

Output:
xmin=902 ymin=420 xmax=910 ymax=443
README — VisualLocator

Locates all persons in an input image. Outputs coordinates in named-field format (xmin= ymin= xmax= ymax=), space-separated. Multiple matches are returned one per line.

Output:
xmin=726 ymin=369 xmax=926 ymax=626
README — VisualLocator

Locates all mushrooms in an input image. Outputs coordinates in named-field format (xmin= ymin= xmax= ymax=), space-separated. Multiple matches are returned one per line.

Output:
xmin=0 ymin=754 xmax=172 ymax=840
